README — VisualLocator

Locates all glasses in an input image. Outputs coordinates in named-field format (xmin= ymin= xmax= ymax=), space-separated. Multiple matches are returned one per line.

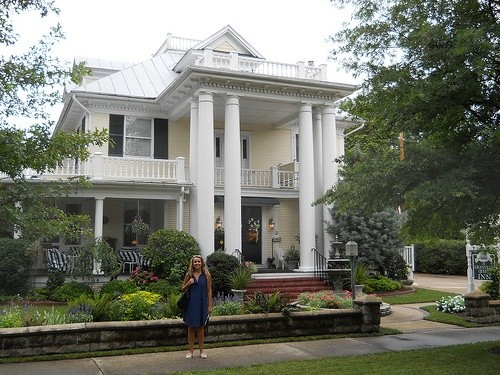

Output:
xmin=192 ymin=255 xmax=202 ymax=258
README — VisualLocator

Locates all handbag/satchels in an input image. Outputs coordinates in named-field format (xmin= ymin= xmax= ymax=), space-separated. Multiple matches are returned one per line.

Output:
xmin=176 ymin=278 xmax=190 ymax=309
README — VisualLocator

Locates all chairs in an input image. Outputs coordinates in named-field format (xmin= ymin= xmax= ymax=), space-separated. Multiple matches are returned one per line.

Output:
xmin=117 ymin=249 xmax=151 ymax=273
xmin=44 ymin=245 xmax=84 ymax=278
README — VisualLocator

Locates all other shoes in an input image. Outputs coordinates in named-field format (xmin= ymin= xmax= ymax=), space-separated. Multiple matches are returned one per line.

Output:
xmin=185 ymin=352 xmax=194 ymax=359
xmin=200 ymin=352 xmax=208 ymax=359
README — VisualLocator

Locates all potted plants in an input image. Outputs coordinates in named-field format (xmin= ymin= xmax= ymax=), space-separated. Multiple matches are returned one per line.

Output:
xmin=349 ymin=262 xmax=370 ymax=296
xmin=284 ymin=245 xmax=300 ymax=271
xmin=332 ymin=264 xmax=345 ymax=292
xmin=225 ymin=264 xmax=253 ymax=302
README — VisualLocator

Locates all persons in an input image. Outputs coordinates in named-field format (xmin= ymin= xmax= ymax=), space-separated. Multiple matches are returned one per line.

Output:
xmin=181 ymin=255 xmax=212 ymax=358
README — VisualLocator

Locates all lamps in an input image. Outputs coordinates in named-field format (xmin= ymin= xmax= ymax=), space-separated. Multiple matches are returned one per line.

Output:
xmin=269 ymin=218 xmax=276 ymax=230
xmin=124 ymin=201 xmax=151 ymax=237
xmin=216 ymin=216 xmax=223 ymax=229
